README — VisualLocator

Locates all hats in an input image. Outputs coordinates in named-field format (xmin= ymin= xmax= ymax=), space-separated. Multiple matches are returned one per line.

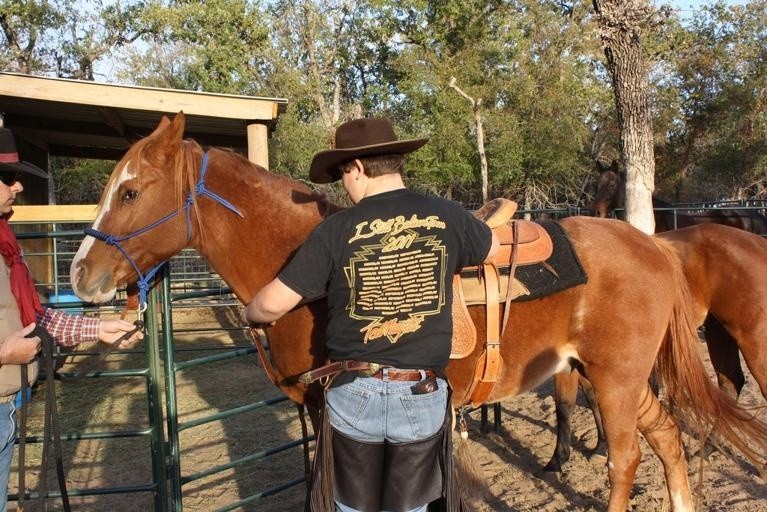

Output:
xmin=1 ymin=128 xmax=49 ymax=181
xmin=309 ymin=119 xmax=429 ymax=183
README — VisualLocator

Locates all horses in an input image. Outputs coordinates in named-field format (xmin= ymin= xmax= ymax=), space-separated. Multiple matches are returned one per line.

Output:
xmin=592 ymin=154 xmax=767 ymax=235
xmin=68 ymin=109 xmax=767 ymax=511
xmin=540 ymin=219 xmax=767 ymax=478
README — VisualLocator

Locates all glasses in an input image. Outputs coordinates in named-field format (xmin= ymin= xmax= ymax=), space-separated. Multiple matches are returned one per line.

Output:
xmin=0 ymin=169 xmax=26 ymax=187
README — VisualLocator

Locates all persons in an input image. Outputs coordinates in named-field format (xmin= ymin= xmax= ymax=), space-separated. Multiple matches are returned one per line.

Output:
xmin=245 ymin=118 xmax=498 ymax=512
xmin=0 ymin=120 xmax=144 ymax=512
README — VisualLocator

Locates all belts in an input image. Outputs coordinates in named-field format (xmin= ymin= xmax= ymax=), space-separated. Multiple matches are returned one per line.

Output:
xmin=298 ymin=360 xmax=434 ymax=384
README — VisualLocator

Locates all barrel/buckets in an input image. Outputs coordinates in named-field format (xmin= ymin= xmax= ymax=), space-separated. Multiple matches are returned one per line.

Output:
xmin=16 ymin=387 xmax=32 ymax=408
xmin=50 ymin=289 xmax=83 ymax=318
